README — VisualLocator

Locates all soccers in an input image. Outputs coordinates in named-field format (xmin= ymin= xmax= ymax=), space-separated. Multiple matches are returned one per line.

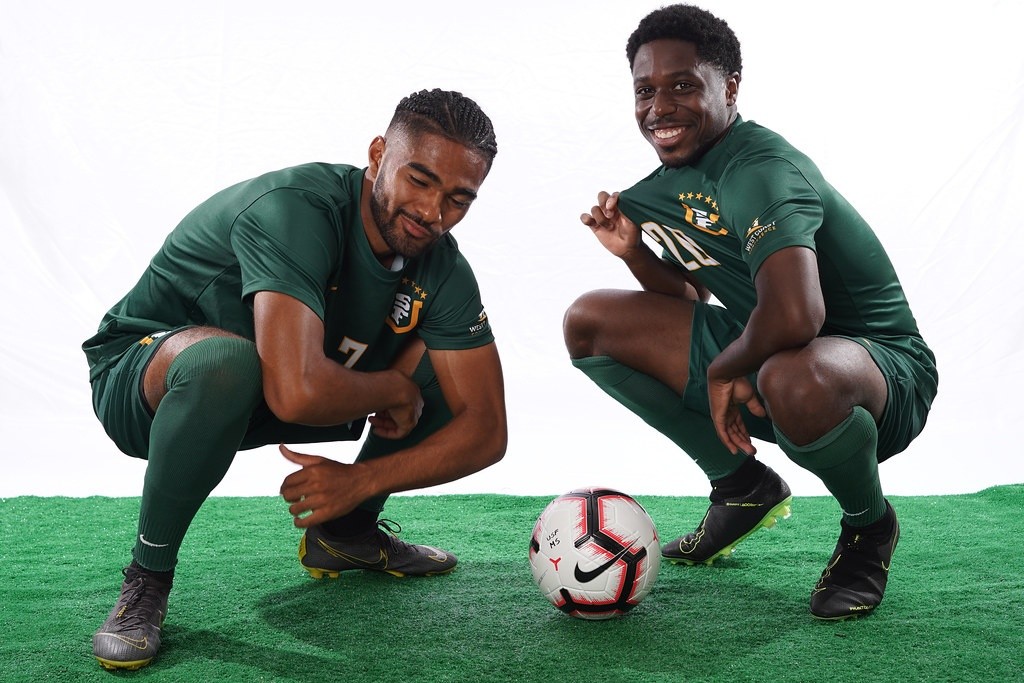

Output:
xmin=529 ymin=490 xmax=659 ymax=620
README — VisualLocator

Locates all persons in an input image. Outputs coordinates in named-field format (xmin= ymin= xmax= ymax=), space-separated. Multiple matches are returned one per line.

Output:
xmin=562 ymin=3 xmax=938 ymax=621
xmin=82 ymin=87 xmax=508 ymax=669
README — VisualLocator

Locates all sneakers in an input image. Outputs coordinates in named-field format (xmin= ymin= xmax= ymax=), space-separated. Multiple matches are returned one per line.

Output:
xmin=660 ymin=466 xmax=793 ymax=566
xmin=810 ymin=498 xmax=900 ymax=623
xmin=92 ymin=566 xmax=173 ymax=671
xmin=298 ymin=520 xmax=459 ymax=579
